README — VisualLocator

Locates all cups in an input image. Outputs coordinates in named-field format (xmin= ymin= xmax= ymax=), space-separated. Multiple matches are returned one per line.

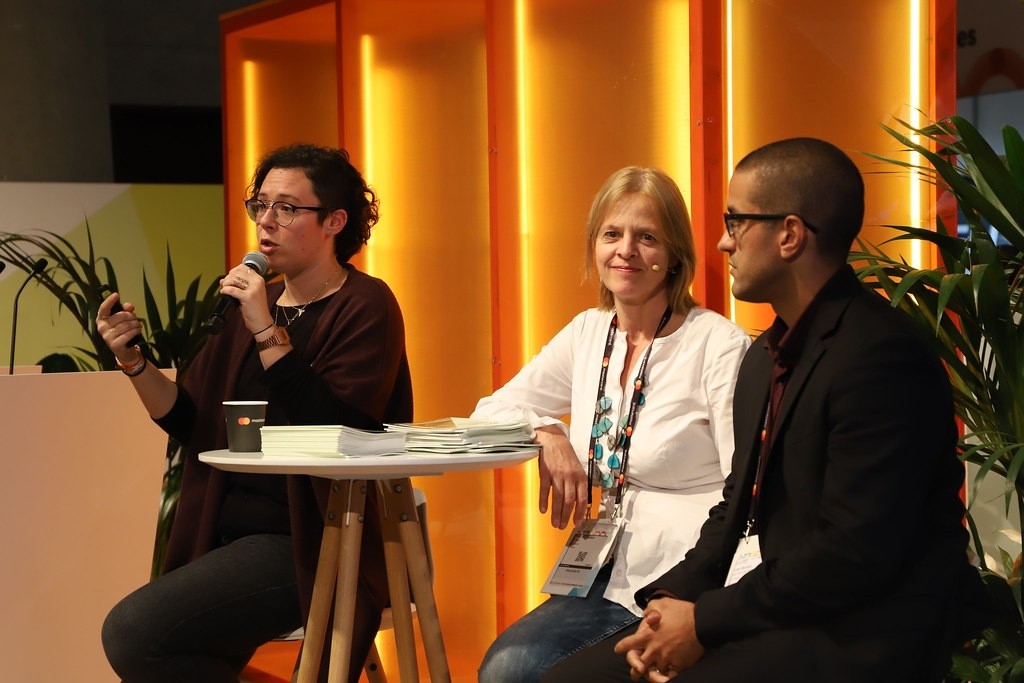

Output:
xmin=222 ymin=400 xmax=269 ymax=452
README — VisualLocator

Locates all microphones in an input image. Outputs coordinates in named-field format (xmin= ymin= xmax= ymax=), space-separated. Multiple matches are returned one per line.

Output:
xmin=208 ymin=251 xmax=270 ymax=335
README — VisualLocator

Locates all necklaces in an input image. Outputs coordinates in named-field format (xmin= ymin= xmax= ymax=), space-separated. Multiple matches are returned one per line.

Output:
xmin=275 ymin=276 xmax=329 ymax=327
xmin=593 ymin=358 xmax=648 ymax=493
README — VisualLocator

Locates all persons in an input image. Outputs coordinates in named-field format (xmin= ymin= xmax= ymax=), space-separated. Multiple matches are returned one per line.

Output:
xmin=96 ymin=144 xmax=414 ymax=683
xmin=616 ymin=136 xmax=970 ymax=683
xmin=469 ymin=164 xmax=762 ymax=683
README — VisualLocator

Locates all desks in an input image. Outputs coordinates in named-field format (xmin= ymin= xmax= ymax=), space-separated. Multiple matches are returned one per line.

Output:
xmin=199 ymin=443 xmax=540 ymax=683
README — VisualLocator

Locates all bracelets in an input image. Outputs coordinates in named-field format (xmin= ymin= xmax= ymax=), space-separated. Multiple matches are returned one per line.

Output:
xmin=254 ymin=321 xmax=274 ymax=335
xmin=114 ymin=346 xmax=146 ymax=376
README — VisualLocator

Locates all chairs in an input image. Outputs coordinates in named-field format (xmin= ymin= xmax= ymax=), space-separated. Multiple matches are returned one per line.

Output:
xmin=270 ymin=488 xmax=435 ymax=683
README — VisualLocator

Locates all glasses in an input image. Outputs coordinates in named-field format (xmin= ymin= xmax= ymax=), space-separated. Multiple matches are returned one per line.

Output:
xmin=721 ymin=211 xmax=819 ymax=237
xmin=244 ymin=195 xmax=333 ymax=229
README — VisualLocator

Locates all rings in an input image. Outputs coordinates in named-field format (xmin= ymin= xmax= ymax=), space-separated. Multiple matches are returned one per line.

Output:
xmin=234 ymin=277 xmax=248 ymax=289
xmin=247 ymin=267 xmax=251 ymax=273
xmin=98 ymin=312 xmax=107 ymax=318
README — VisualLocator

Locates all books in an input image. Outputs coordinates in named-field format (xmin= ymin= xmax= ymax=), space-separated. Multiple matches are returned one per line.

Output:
xmin=260 ymin=417 xmax=542 ymax=459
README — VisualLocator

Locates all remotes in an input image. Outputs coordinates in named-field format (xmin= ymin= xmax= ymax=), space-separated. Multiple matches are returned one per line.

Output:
xmin=98 ymin=285 xmax=142 ymax=349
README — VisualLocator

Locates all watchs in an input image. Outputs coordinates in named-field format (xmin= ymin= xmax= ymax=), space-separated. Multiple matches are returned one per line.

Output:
xmin=257 ymin=328 xmax=290 ymax=351
